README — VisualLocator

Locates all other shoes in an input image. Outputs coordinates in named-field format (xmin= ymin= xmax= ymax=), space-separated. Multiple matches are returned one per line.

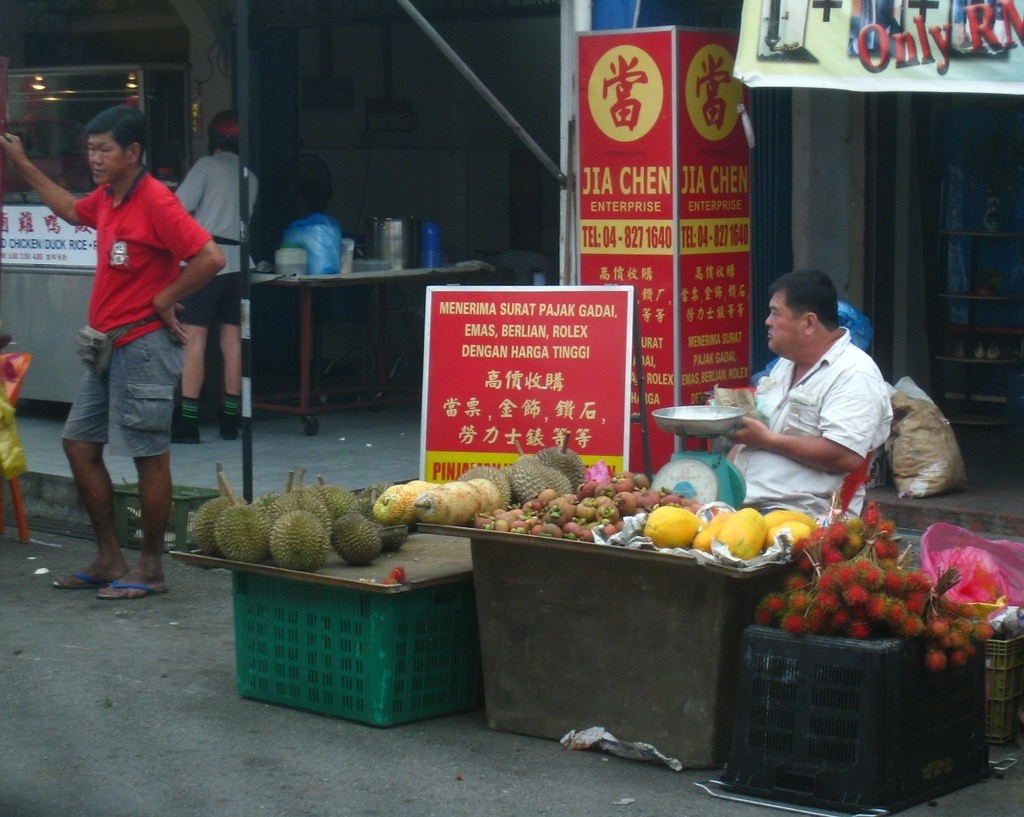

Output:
xmin=220 ymin=422 xmax=238 ymax=440
xmin=171 ymin=424 xmax=200 ymax=445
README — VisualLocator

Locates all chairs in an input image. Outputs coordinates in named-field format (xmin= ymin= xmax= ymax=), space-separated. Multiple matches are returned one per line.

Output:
xmin=0 ymin=351 xmax=33 ymax=544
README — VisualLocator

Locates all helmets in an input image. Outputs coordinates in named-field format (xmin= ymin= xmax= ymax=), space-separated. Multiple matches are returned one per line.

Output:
xmin=209 ymin=110 xmax=241 ymax=147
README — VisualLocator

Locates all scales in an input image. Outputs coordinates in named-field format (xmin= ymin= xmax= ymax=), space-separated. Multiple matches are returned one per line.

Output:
xmin=650 ymin=403 xmax=747 ymax=511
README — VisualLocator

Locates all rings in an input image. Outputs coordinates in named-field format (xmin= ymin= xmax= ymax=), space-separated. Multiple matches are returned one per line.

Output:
xmin=5 ymin=133 xmax=10 ymax=140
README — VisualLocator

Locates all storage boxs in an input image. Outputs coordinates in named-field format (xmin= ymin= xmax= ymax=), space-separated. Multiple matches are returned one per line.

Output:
xmin=985 ymin=634 xmax=1024 ymax=744
xmin=866 ymin=444 xmax=887 ymax=490
xmin=722 ymin=624 xmax=988 ymax=817
xmin=112 ymin=483 xmax=219 ymax=553
xmin=233 ymin=572 xmax=484 ymax=727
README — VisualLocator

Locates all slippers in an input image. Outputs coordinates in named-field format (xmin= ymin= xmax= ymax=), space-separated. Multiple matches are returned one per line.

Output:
xmin=52 ymin=572 xmax=110 ymax=589
xmin=96 ymin=579 xmax=168 ymax=600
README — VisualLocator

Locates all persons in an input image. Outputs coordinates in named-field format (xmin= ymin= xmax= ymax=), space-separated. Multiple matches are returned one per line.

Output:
xmin=146 ymin=140 xmax=182 ymax=194
xmin=725 ymin=268 xmax=894 ymax=522
xmin=168 ymin=109 xmax=259 ymax=445
xmin=0 ymin=104 xmax=228 ymax=601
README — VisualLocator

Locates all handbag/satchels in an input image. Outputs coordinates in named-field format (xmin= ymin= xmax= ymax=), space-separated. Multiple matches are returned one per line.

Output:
xmin=76 ymin=325 xmax=111 ymax=374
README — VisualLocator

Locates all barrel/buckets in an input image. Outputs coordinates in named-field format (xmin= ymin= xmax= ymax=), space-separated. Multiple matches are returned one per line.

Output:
xmin=372 ymin=217 xmax=414 ymax=271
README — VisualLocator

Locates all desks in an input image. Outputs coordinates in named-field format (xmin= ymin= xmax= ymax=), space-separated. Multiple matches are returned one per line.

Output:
xmin=216 ymin=266 xmax=491 ymax=436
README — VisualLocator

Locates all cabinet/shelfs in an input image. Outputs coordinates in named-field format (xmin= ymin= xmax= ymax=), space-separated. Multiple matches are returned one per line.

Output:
xmin=935 ymin=180 xmax=1024 ymax=425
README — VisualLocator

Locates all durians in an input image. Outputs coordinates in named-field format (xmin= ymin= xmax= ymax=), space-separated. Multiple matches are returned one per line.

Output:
xmin=192 ymin=460 xmax=409 ymax=572
xmin=459 ymin=430 xmax=586 ymax=508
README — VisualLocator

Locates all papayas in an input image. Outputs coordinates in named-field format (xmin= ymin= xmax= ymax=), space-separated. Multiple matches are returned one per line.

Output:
xmin=643 ymin=505 xmax=816 ymax=562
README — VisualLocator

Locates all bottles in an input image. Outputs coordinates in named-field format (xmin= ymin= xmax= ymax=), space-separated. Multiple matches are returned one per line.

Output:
xmin=274 ymin=244 xmax=307 ymax=276
xmin=419 ymin=219 xmax=442 ymax=268
